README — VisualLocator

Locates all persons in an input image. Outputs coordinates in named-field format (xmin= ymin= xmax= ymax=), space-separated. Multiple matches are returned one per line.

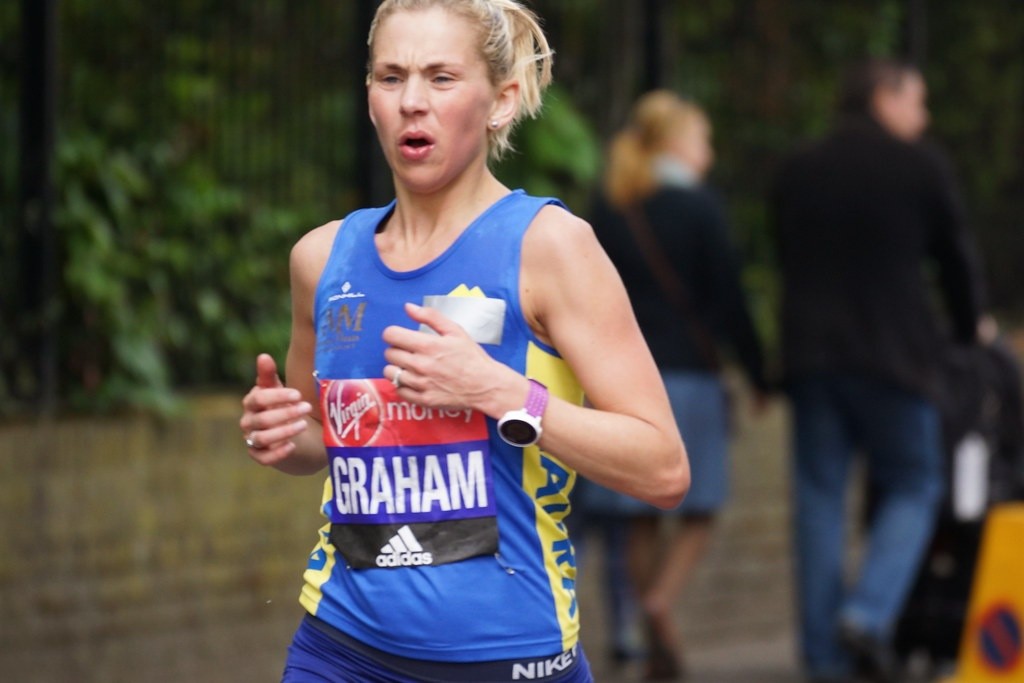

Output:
xmin=237 ymin=0 xmax=691 ymax=683
xmin=576 ymin=92 xmax=773 ymax=683
xmin=771 ymin=59 xmax=998 ymax=683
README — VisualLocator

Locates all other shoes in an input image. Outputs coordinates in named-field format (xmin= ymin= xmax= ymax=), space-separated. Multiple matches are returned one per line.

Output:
xmin=639 ymin=609 xmax=685 ymax=683
xmin=832 ymin=615 xmax=899 ymax=683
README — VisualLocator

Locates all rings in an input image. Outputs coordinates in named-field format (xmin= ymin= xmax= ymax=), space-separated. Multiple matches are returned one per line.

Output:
xmin=246 ymin=434 xmax=254 ymax=447
xmin=392 ymin=371 xmax=401 ymax=389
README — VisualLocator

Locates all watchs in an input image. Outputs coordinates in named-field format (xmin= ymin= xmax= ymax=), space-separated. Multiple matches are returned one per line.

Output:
xmin=498 ymin=378 xmax=548 ymax=447
